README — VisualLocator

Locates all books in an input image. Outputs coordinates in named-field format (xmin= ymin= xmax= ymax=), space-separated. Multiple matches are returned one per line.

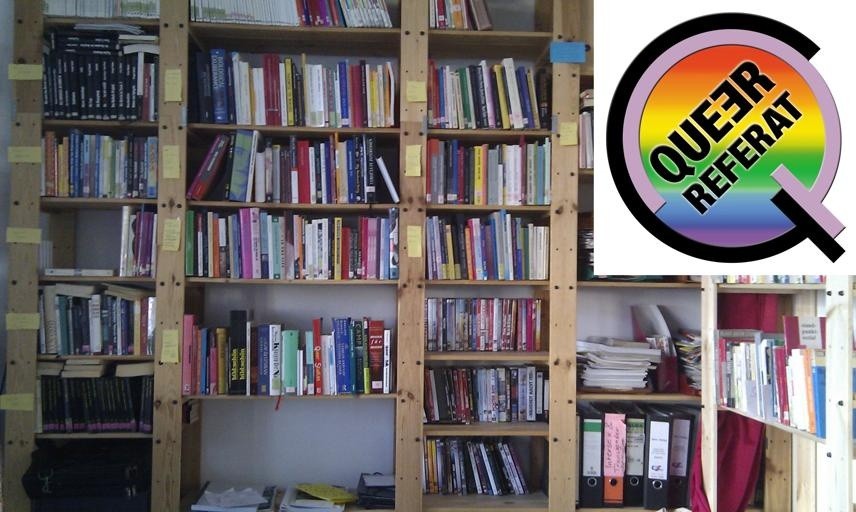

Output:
xmin=188 ymin=1 xmax=550 ymax=278
xmin=185 ymin=468 xmax=394 ymax=511
xmin=714 ymin=314 xmax=826 ymax=440
xmin=574 ymin=326 xmax=702 ymax=394
xmin=40 ymin=1 xmax=159 ymax=512
xmin=183 ymin=306 xmax=396 ymax=394
xmin=676 ymin=275 xmax=827 ymax=284
xmin=424 ymin=297 xmax=549 ymax=496
